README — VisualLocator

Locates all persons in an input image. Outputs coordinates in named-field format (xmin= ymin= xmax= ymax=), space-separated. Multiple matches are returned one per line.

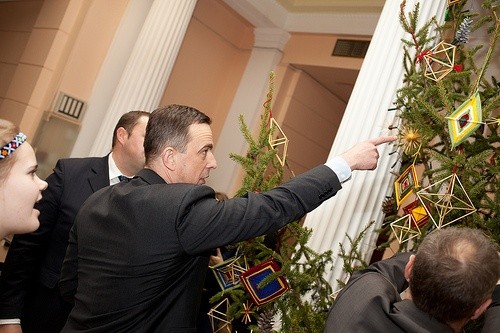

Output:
xmin=0 ymin=118 xmax=48 ymax=259
xmin=1 ymin=111 xmax=150 ymax=333
xmin=59 ymin=105 xmax=398 ymax=333
xmin=323 ymin=226 xmax=500 ymax=333
xmin=208 ymin=193 xmax=239 ymax=299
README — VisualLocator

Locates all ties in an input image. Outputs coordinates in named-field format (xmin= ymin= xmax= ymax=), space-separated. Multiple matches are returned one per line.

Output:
xmin=119 ymin=176 xmax=130 ymax=183
